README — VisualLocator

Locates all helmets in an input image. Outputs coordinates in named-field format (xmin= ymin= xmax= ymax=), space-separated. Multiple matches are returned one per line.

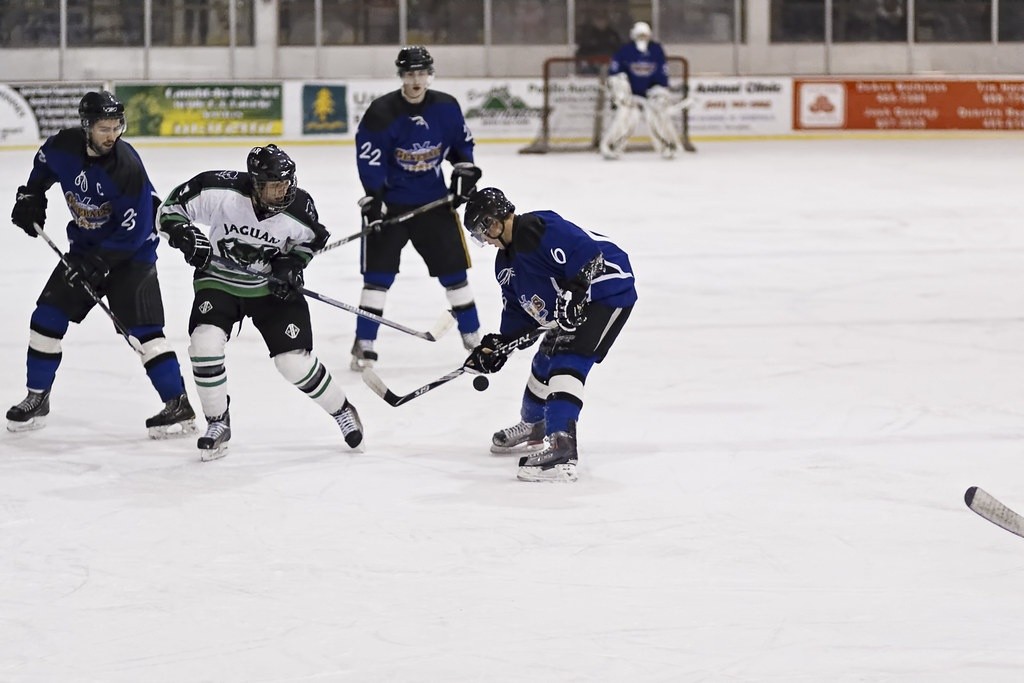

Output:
xmin=629 ymin=21 xmax=652 ymax=52
xmin=395 ymin=47 xmax=434 ymax=69
xmin=463 ymin=187 xmax=515 ymax=239
xmin=78 ymin=91 xmax=125 ymax=122
xmin=247 ymin=143 xmax=297 ymax=212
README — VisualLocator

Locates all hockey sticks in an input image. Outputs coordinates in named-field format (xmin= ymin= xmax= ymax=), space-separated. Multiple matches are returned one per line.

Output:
xmin=313 ymin=194 xmax=457 ymax=257
xmin=596 ymin=82 xmax=694 ymax=117
xmin=208 ymin=253 xmax=457 ymax=342
xmin=965 ymin=485 xmax=1023 ymax=538
xmin=32 ymin=221 xmax=148 ymax=356
xmin=361 ymin=319 xmax=561 ymax=407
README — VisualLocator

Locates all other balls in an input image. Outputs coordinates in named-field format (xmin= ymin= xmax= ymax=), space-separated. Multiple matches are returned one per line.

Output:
xmin=473 ymin=376 xmax=489 ymax=391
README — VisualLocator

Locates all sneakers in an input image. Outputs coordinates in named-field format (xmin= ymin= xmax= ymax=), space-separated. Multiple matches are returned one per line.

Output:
xmin=489 ymin=419 xmax=545 ymax=455
xmin=197 ymin=395 xmax=230 ymax=462
xmin=462 ymin=335 xmax=484 ymax=351
xmin=330 ymin=397 xmax=367 ymax=453
xmin=146 ymin=394 xmax=196 ymax=438
xmin=6 ymin=390 xmax=50 ymax=433
xmin=350 ymin=339 xmax=377 ymax=370
xmin=517 ymin=432 xmax=578 ymax=481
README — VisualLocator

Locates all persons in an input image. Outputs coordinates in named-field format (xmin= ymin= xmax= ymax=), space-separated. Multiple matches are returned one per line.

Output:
xmin=465 ymin=187 xmax=639 ymax=473
xmin=159 ymin=143 xmax=364 ymax=450
xmin=351 ymin=46 xmax=482 ymax=360
xmin=575 ymin=10 xmax=623 ymax=75
xmin=600 ymin=25 xmax=681 ymax=161
xmin=6 ymin=87 xmax=196 ymax=427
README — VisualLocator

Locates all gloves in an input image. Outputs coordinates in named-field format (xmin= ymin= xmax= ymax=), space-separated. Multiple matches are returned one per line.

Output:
xmin=10 ymin=185 xmax=48 ymax=238
xmin=463 ymin=338 xmax=508 ymax=374
xmin=359 ymin=196 xmax=388 ymax=229
xmin=268 ymin=268 xmax=304 ymax=300
xmin=170 ymin=225 xmax=214 ymax=271
xmin=449 ymin=169 xmax=477 ymax=209
xmin=60 ymin=255 xmax=109 ymax=293
xmin=553 ymin=289 xmax=587 ymax=333
